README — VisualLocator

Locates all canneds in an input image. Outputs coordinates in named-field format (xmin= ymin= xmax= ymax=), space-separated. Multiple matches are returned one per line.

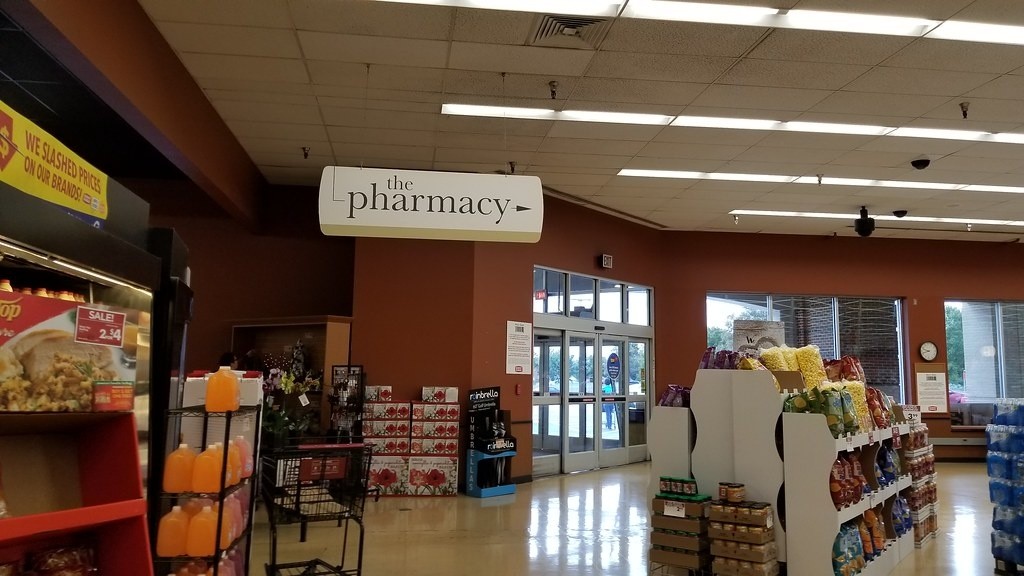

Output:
xmin=651 ymin=476 xmax=778 ymax=571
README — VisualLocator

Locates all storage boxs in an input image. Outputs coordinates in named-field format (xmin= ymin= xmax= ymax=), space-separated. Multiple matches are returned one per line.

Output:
xmin=649 ymin=515 xmax=709 ymax=533
xmin=710 ymin=540 xmax=778 ymax=562
xmin=703 ymin=504 xmax=774 ymax=525
xmin=650 ymin=530 xmax=708 ymax=551
xmin=712 ymin=557 xmax=780 ymax=576
xmin=708 ymin=524 xmax=776 ymax=545
xmin=647 ymin=548 xmax=710 ymax=569
xmin=166 ymin=367 xmax=263 ymax=454
xmin=651 ymin=496 xmax=711 ymax=516
xmin=355 ymin=385 xmax=460 ymax=496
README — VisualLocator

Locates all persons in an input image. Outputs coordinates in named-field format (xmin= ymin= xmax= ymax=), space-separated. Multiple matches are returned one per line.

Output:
xmin=219 ymin=349 xmax=255 ymax=371
xmin=601 ymin=379 xmax=614 ymax=430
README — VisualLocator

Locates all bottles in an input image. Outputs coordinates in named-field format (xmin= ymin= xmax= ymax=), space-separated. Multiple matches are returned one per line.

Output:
xmin=900 ymin=422 xmax=938 ymax=543
xmin=152 ymin=366 xmax=264 ymax=576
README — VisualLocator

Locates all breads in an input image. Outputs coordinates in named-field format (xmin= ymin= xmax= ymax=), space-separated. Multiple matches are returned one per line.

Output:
xmin=0 ymin=329 xmax=120 ymax=386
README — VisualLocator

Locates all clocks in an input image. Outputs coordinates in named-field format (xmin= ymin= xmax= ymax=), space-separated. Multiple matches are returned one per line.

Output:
xmin=919 ymin=341 xmax=938 ymax=361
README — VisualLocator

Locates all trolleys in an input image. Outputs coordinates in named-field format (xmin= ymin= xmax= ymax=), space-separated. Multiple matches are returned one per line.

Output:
xmin=260 ymin=436 xmax=378 ymax=576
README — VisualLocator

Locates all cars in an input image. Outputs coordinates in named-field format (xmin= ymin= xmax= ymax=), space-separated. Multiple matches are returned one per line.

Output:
xmin=534 ymin=379 xmax=645 ymax=396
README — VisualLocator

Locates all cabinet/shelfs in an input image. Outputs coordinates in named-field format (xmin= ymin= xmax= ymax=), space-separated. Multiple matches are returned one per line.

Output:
xmin=0 ymin=404 xmax=155 ymax=576
xmin=643 ymin=370 xmax=939 ymax=576
xmin=230 ymin=312 xmax=354 ymax=444
